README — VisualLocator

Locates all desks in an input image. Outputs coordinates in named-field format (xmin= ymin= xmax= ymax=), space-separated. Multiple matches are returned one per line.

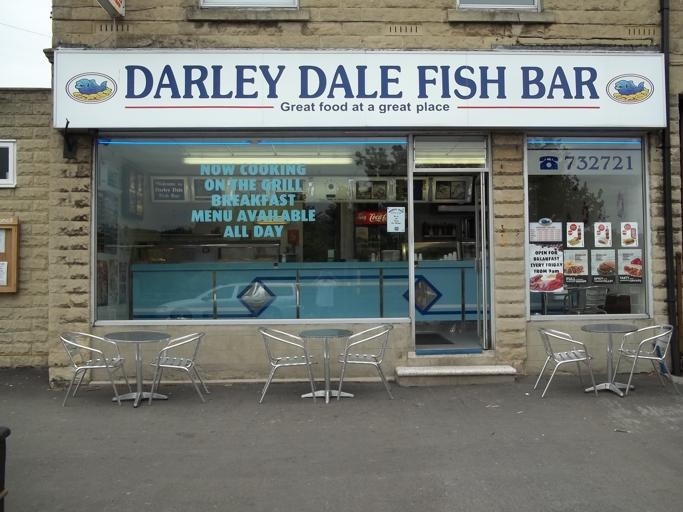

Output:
xmin=104 ymin=332 xmax=170 ymax=408
xmin=294 ymin=329 xmax=357 ymax=402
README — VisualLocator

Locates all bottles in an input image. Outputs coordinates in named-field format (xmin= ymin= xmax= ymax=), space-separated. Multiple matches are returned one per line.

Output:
xmin=605 ymin=226 xmax=609 ymax=239
xmin=578 ymin=225 xmax=581 ymax=240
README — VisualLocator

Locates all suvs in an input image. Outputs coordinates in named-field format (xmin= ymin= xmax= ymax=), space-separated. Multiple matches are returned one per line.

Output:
xmin=159 ymin=283 xmax=303 ymax=328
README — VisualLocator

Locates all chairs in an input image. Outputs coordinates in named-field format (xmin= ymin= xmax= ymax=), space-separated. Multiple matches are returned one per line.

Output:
xmin=57 ymin=330 xmax=132 ymax=408
xmin=256 ymin=326 xmax=318 ymax=403
xmin=149 ymin=332 xmax=213 ymax=406
xmin=532 ymin=286 xmax=680 ymax=398
xmin=333 ymin=323 xmax=394 ymax=404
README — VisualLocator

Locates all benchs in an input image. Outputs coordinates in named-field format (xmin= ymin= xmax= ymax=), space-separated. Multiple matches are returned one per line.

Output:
xmin=128 ymin=241 xmax=478 ymax=330
